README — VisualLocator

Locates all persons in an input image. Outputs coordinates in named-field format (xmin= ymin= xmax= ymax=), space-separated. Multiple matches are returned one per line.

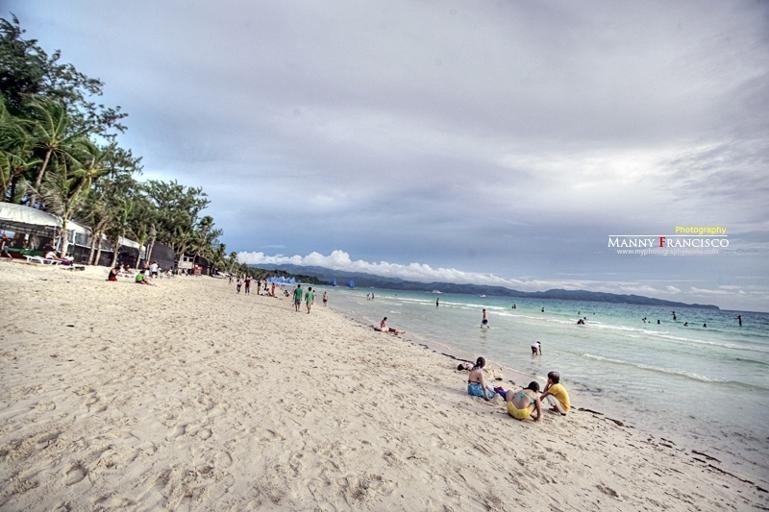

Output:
xmin=435 ymin=297 xmax=439 ymax=308
xmin=531 ymin=340 xmax=543 ymax=356
xmin=62 ymin=253 xmax=75 ymax=266
xmin=228 ymin=273 xmax=329 ymax=314
xmin=45 ymin=249 xmax=62 ymax=261
xmin=539 ymin=371 xmax=570 ymax=415
xmin=136 ymin=270 xmax=154 ymax=286
xmin=150 ymin=260 xmax=158 ymax=279
xmin=506 ymin=381 xmax=543 ymax=421
xmin=480 ymin=308 xmax=489 ymax=328
xmin=466 ymin=356 xmax=496 ymax=401
xmin=380 ymin=316 xmax=396 ymax=332
xmin=105 ymin=266 xmax=121 ymax=282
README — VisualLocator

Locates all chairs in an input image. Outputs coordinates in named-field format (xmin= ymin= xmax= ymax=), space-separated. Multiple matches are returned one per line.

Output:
xmin=24 ymin=254 xmax=62 ymax=266
